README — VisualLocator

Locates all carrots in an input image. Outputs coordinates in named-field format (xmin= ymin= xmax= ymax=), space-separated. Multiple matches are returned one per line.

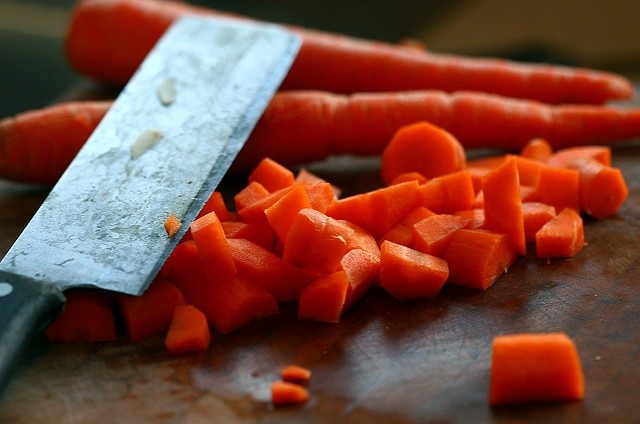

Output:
xmin=60 ymin=0 xmax=637 ymax=104
xmin=3 ymin=86 xmax=640 ymax=181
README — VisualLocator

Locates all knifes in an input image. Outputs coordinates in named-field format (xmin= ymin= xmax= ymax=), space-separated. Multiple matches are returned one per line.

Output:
xmin=0 ymin=12 xmax=304 ymax=396
